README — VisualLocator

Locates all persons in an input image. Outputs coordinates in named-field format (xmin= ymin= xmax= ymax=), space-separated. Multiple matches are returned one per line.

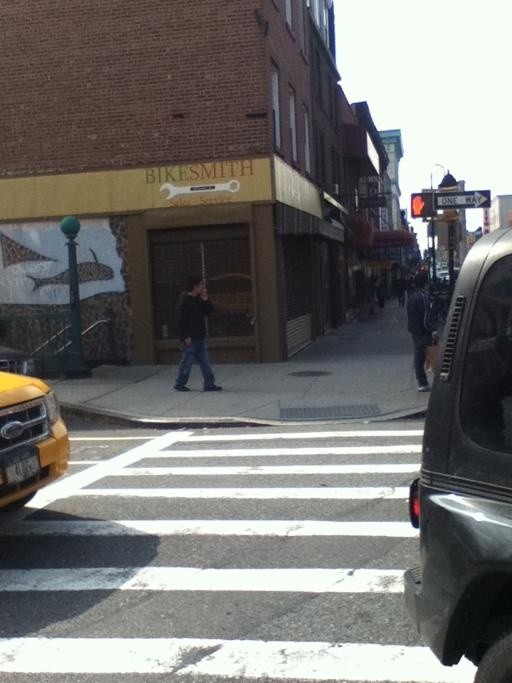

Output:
xmin=173 ymin=273 xmax=223 ymax=391
xmin=406 ymin=272 xmax=438 ymax=392
xmin=356 ymin=267 xmax=457 ymax=313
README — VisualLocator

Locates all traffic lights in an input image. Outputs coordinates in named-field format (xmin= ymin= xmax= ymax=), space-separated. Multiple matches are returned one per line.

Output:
xmin=409 ymin=191 xmax=434 ymax=217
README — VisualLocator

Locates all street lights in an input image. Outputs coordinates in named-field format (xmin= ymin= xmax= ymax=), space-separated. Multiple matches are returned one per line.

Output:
xmin=56 ymin=215 xmax=84 ymax=381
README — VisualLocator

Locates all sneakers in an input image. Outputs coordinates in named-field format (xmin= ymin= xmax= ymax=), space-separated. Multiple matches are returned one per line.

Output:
xmin=174 ymin=383 xmax=223 ymax=391
xmin=416 ymin=376 xmax=429 ymax=391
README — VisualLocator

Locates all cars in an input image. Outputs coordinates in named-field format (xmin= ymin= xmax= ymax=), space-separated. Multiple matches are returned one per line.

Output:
xmin=403 ymin=223 xmax=512 ymax=682
xmin=0 ymin=370 xmax=70 ymax=516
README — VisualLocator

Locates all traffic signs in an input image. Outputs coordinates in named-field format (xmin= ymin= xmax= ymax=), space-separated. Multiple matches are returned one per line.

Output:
xmin=434 ymin=189 xmax=492 ymax=208
